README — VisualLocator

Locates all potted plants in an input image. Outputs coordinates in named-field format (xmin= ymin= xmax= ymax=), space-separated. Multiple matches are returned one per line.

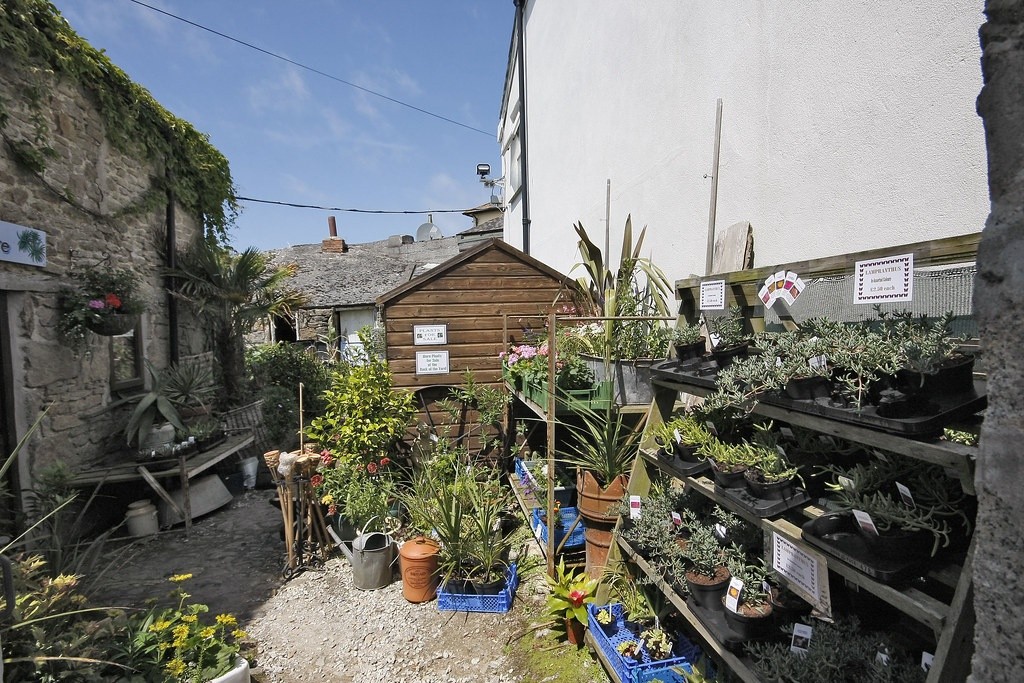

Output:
xmin=78 ymin=370 xmax=183 ymax=453
xmin=520 ymin=213 xmax=988 ymax=683
xmin=171 ymin=362 xmax=222 ymax=430
xmin=336 ymin=431 xmax=513 ymax=597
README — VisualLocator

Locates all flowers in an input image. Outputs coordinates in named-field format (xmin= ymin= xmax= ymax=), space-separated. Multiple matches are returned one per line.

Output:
xmin=546 ymin=496 xmax=562 ymax=529
xmin=55 ymin=269 xmax=146 ymax=361
xmin=103 ymin=562 xmax=251 ymax=683
xmin=514 ymin=456 xmax=564 ymax=497
xmin=498 ymin=317 xmax=607 ymax=374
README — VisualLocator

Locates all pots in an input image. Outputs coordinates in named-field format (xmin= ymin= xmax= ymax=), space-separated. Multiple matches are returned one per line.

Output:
xmin=159 ymin=474 xmax=232 ymax=529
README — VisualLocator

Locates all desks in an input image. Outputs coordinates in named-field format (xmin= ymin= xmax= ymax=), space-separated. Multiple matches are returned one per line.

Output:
xmin=65 ymin=434 xmax=256 ymax=534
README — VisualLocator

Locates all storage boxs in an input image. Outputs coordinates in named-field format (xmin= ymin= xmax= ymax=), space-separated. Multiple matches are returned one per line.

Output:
xmin=530 ymin=506 xmax=584 ymax=551
xmin=436 ymin=563 xmax=520 ymax=613
xmin=501 ymin=364 xmax=616 ymax=413
xmin=586 ymin=602 xmax=700 ymax=683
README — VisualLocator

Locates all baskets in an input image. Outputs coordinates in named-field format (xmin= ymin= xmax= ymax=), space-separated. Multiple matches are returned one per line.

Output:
xmin=262 ymin=444 xmax=321 ymax=481
xmin=219 ymin=398 xmax=296 ymax=487
xmin=84 ymin=313 xmax=139 ymax=336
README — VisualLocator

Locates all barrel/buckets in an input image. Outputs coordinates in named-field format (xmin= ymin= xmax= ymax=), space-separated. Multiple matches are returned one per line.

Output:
xmin=235 ymin=456 xmax=259 ymax=487
xmin=400 ymin=536 xmax=440 ymax=602
xmin=125 ymin=499 xmax=158 ymax=536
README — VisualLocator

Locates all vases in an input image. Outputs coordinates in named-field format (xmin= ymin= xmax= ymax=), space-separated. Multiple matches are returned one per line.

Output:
xmin=522 ymin=458 xmax=576 ymax=509
xmin=212 ymin=650 xmax=249 ymax=683
xmin=85 ymin=308 xmax=139 ymax=338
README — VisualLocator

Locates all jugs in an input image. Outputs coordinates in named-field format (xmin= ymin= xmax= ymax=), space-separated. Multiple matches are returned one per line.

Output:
xmin=325 ymin=515 xmax=401 ymax=590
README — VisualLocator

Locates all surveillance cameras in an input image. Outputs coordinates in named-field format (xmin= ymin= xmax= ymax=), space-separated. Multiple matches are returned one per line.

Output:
xmin=477 ymin=163 xmax=491 ymax=175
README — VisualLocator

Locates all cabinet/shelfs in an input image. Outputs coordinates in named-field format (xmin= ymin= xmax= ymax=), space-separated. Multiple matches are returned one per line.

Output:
xmin=499 ymin=313 xmax=676 ymax=584
xmin=584 ymin=231 xmax=982 ymax=683
